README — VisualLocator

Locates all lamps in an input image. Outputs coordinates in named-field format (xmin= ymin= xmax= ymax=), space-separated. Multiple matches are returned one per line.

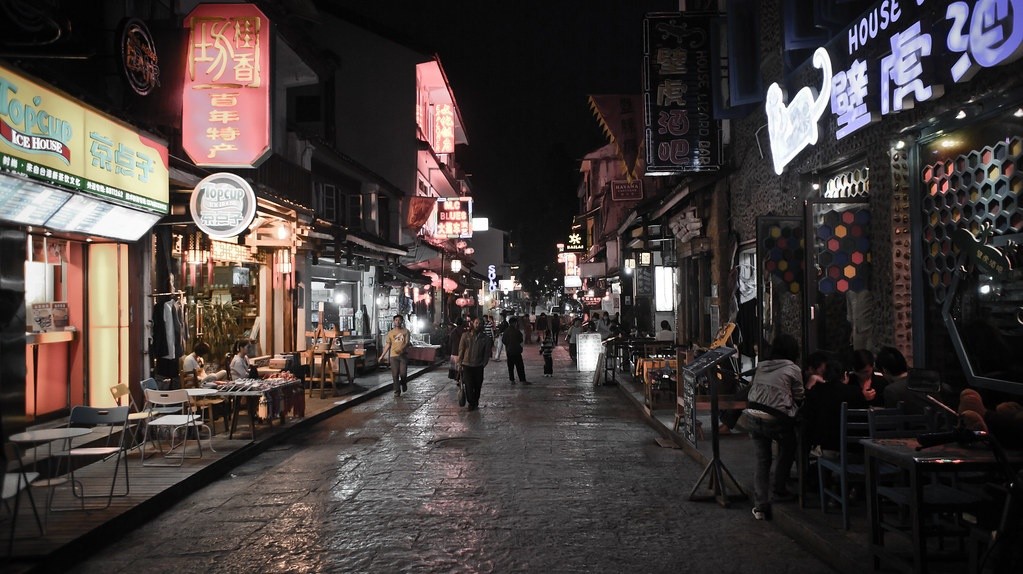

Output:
xmin=310 ymin=244 xmax=365 ymax=267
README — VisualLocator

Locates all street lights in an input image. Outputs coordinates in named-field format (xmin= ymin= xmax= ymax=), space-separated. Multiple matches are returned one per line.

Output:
xmin=441 ymin=251 xmax=465 ymax=326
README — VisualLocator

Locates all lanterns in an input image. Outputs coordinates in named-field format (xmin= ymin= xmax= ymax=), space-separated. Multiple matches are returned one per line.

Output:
xmin=425 ymin=271 xmax=457 ymax=293
xmin=456 ymin=298 xmax=470 ymax=307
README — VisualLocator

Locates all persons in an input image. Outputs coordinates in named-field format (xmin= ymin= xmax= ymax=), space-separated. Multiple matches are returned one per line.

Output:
xmin=502 ymin=317 xmax=531 ymax=385
xmin=588 ymin=312 xmax=619 ymax=340
xmin=229 ymin=338 xmax=260 ymax=401
xmin=447 ymin=313 xmax=508 ymax=389
xmin=538 ymin=329 xmax=555 ymax=377
xmin=535 ymin=313 xmax=560 ymax=345
xmin=183 ymin=341 xmax=227 ymax=401
xmin=565 ymin=318 xmax=584 ymax=361
xmin=743 ymin=333 xmax=805 ymax=520
xmin=458 ymin=318 xmax=493 ymax=410
xmin=794 ymin=347 xmax=1023 ymax=508
xmin=377 ymin=315 xmax=409 ymax=397
xmin=656 ymin=320 xmax=673 ymax=341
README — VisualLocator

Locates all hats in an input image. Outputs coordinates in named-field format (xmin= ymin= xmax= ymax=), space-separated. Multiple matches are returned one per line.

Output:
xmin=455 ymin=318 xmax=465 ymax=324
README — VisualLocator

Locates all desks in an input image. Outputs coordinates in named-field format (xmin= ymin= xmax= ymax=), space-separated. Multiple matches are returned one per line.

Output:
xmin=165 ymin=387 xmax=219 ymax=456
xmin=300 ymin=352 xmax=363 ymax=392
xmin=210 ymin=377 xmax=299 ymax=440
xmin=7 ymin=426 xmax=92 ymax=529
xmin=406 ymin=344 xmax=441 ymax=366
xmin=861 ymin=434 xmax=1023 ymax=573
xmin=636 ymin=357 xmax=676 ymax=396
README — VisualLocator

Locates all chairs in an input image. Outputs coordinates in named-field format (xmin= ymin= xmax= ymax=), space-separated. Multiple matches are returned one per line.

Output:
xmin=789 ymin=396 xmax=1023 ymax=574
xmin=636 ymin=340 xmax=676 ymax=417
xmin=0 ymin=346 xmax=311 ymax=557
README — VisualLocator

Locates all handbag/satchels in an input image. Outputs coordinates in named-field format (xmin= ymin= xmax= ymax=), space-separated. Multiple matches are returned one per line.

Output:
xmin=456 ymin=364 xmax=466 ymax=407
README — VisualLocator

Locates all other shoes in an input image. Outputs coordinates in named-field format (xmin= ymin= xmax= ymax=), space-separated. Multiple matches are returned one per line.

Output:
xmin=394 ymin=391 xmax=400 ymax=397
xmin=469 ymin=401 xmax=474 ymax=411
xmin=511 ymin=380 xmax=516 ymax=385
xmin=521 ymin=381 xmax=531 ymax=385
xmin=402 ymin=384 xmax=407 ymax=392
xmin=544 ymin=373 xmax=552 ymax=378
xmin=752 ymin=507 xmax=766 ymax=520
xmin=829 ymin=499 xmax=838 ymax=508
xmin=474 ymin=400 xmax=478 ymax=407
xmin=849 ymin=487 xmax=861 ymax=499
